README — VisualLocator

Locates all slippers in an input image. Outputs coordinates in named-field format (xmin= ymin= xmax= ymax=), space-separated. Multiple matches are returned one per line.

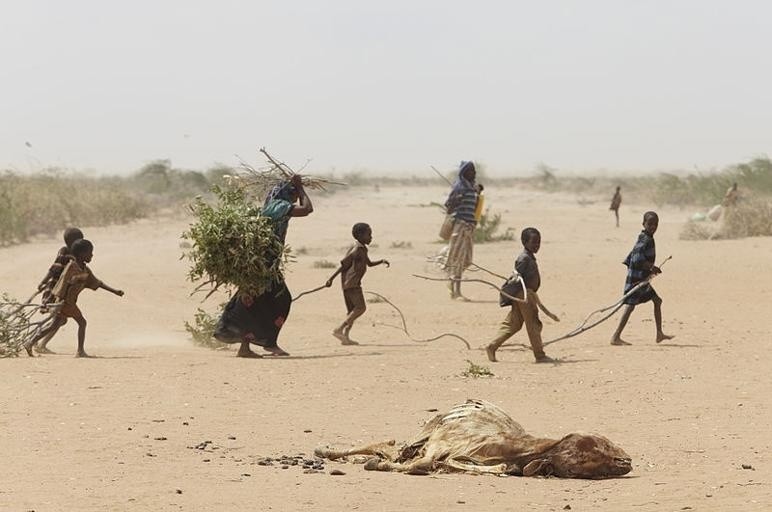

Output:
xmin=262 ymin=349 xmax=291 ymax=359
xmin=332 ymin=331 xmax=347 ymax=343
xmin=236 ymin=351 xmax=262 ymax=357
xmin=74 ymin=351 xmax=92 ymax=358
xmin=39 ymin=348 xmax=56 ymax=354
xmin=23 ymin=345 xmax=32 ymax=356
xmin=449 ymin=293 xmax=471 ymax=302
xmin=343 ymin=341 xmax=359 ymax=345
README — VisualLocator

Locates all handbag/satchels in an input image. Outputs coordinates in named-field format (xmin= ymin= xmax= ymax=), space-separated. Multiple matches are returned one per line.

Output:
xmin=440 ymin=210 xmax=456 ymax=240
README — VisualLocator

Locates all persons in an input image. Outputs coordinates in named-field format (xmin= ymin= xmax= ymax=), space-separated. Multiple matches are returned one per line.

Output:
xmin=436 ymin=159 xmax=483 ymax=302
xmin=724 ymin=183 xmax=738 ymax=205
xmin=325 ymin=223 xmax=390 ymax=345
xmin=611 ymin=210 xmax=675 ymax=346
xmin=37 ymin=227 xmax=84 ymax=354
xmin=611 ymin=186 xmax=621 ymax=227
xmin=212 ymin=174 xmax=313 ymax=359
xmin=24 ymin=239 xmax=124 ymax=358
xmin=483 ymin=227 xmax=559 ymax=363
